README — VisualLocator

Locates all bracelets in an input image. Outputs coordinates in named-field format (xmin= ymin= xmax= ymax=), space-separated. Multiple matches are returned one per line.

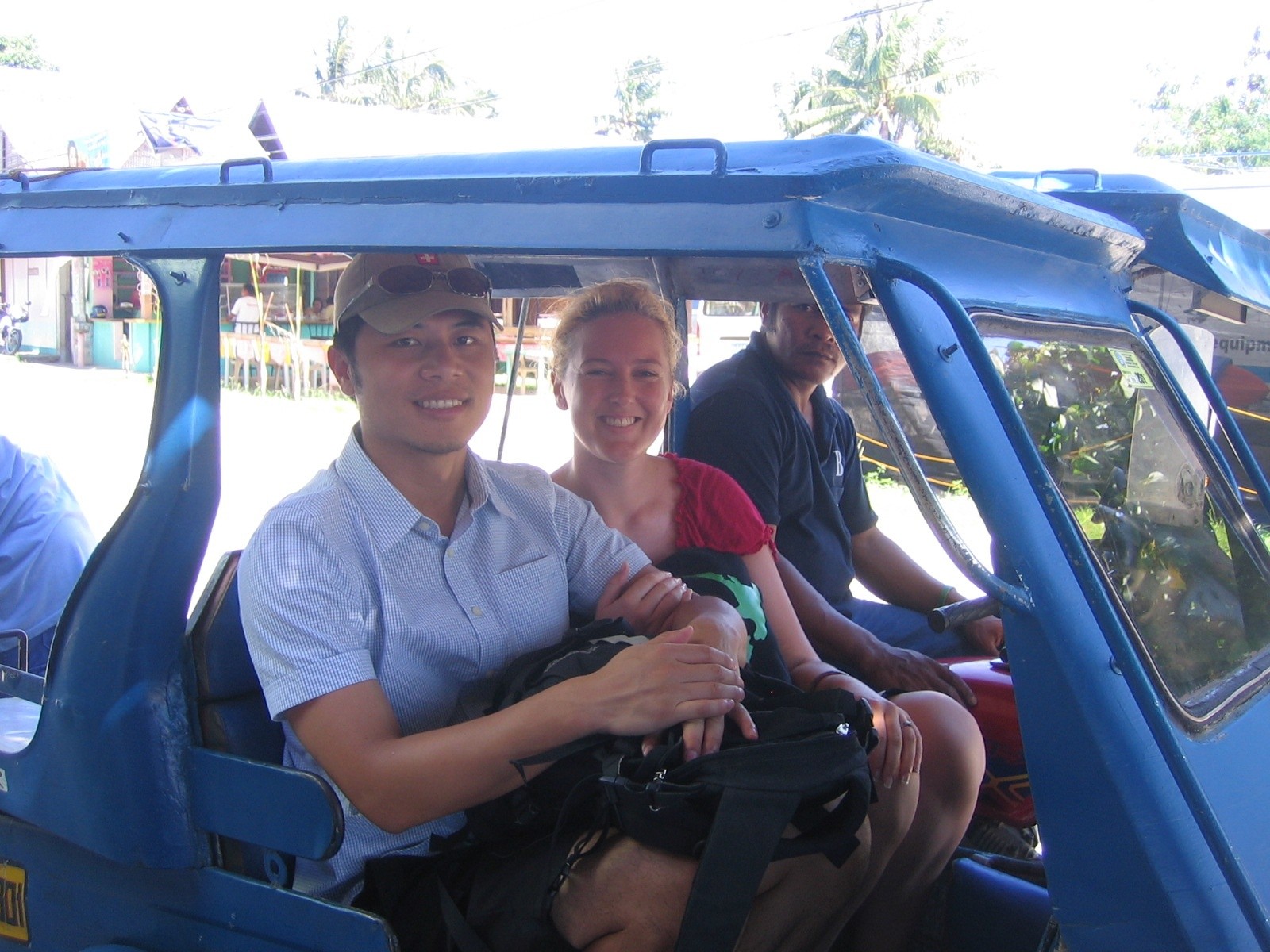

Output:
xmin=808 ymin=671 xmax=848 ymax=693
xmin=936 ymin=585 xmax=955 ymax=608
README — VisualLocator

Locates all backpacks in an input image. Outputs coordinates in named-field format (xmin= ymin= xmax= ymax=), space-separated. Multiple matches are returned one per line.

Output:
xmin=444 ymin=614 xmax=881 ymax=950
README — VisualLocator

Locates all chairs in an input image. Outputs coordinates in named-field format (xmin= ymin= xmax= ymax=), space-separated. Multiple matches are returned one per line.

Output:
xmin=178 ymin=547 xmax=297 ymax=890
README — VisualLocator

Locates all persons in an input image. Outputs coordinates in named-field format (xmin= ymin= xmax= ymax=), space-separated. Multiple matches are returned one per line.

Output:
xmin=238 ymin=251 xmax=874 ymax=952
xmin=543 ymin=278 xmax=984 ymax=952
xmin=228 ymin=283 xmax=260 ymax=390
xmin=0 ymin=375 xmax=99 ymax=699
xmin=302 ymin=296 xmax=336 ymax=323
xmin=672 ymin=256 xmax=1005 ymax=709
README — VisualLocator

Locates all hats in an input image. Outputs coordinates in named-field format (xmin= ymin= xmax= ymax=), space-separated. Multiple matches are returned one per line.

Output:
xmin=331 ymin=246 xmax=506 ymax=334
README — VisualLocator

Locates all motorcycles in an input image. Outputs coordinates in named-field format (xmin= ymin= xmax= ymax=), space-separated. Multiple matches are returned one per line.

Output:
xmin=1 ymin=136 xmax=1270 ymax=952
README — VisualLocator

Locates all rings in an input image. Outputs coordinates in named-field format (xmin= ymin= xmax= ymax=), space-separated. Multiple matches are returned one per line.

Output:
xmin=901 ymin=720 xmax=913 ymax=728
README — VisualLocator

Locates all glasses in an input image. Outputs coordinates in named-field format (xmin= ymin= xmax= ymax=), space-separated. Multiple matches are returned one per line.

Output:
xmin=335 ymin=268 xmax=493 ymax=334
